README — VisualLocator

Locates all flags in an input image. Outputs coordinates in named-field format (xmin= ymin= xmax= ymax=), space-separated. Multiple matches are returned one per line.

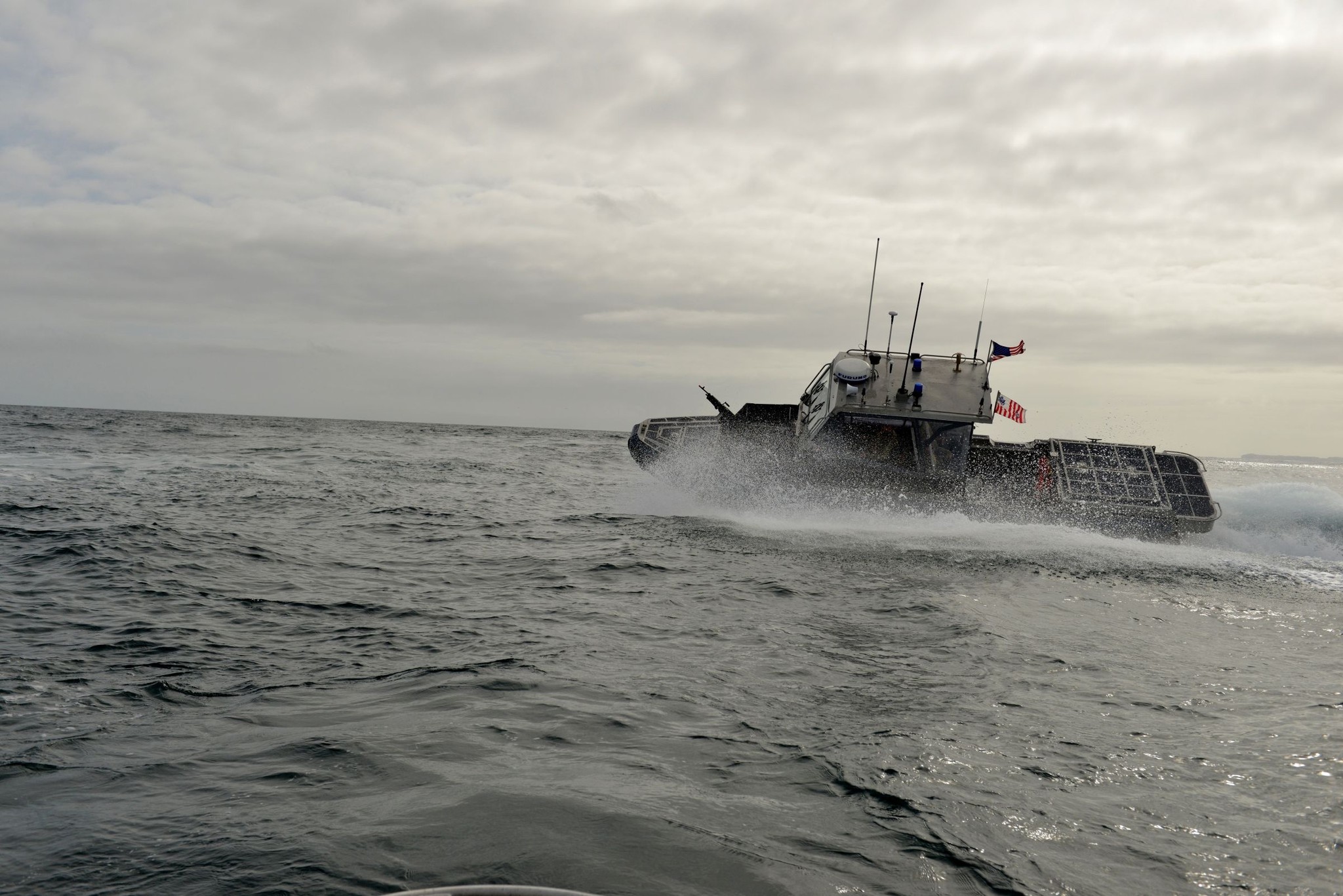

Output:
xmin=994 ymin=390 xmax=1027 ymax=424
xmin=987 ymin=340 xmax=1026 ymax=362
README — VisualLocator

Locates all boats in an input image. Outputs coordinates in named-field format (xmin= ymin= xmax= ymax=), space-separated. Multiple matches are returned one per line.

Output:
xmin=624 ymin=236 xmax=1226 ymax=549
